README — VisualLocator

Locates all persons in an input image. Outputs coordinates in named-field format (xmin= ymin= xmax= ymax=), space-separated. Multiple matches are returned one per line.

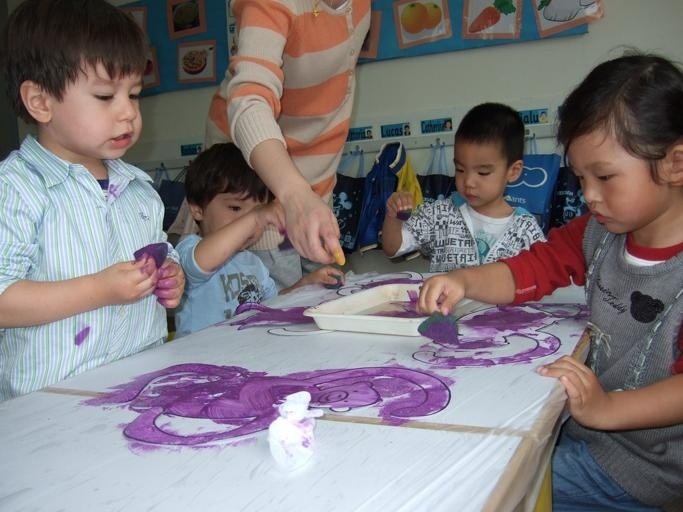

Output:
xmin=416 ymin=54 xmax=683 ymax=511
xmin=404 ymin=125 xmax=410 ymax=135
xmin=382 ymin=103 xmax=548 ymax=273
xmin=173 ymin=140 xmax=345 ymax=341
xmin=165 ymin=0 xmax=372 ymax=296
xmin=367 ymin=130 xmax=372 ymax=139
xmin=197 ymin=146 xmax=202 ymax=153
xmin=445 ymin=120 xmax=452 ymax=130
xmin=0 ymin=0 xmax=187 ymax=408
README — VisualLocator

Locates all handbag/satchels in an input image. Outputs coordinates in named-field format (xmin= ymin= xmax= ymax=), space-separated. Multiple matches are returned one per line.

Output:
xmin=159 ymin=179 xmax=187 ymax=232
xmin=333 ymin=173 xmax=367 ymax=254
xmin=504 ymin=154 xmax=588 ymax=234
xmin=416 ymin=174 xmax=457 ymax=203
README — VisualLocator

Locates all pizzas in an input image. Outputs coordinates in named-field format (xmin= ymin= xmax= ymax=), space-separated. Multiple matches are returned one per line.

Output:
xmin=184 ymin=51 xmax=206 ymax=73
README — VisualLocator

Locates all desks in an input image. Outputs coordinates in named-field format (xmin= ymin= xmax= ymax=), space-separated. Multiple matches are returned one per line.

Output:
xmin=0 ymin=250 xmax=591 ymax=511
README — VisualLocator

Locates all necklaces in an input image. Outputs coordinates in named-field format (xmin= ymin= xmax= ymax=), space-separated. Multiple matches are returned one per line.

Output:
xmin=312 ymin=1 xmax=322 ymax=16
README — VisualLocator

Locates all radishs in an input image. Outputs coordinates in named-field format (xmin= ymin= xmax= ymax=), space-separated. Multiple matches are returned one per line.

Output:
xmin=579 ymin=0 xmax=596 ymax=6
xmin=538 ymin=0 xmax=584 ymax=20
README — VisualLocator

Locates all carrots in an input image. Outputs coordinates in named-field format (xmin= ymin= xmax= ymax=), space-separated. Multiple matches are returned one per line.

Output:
xmin=469 ymin=0 xmax=516 ymax=33
